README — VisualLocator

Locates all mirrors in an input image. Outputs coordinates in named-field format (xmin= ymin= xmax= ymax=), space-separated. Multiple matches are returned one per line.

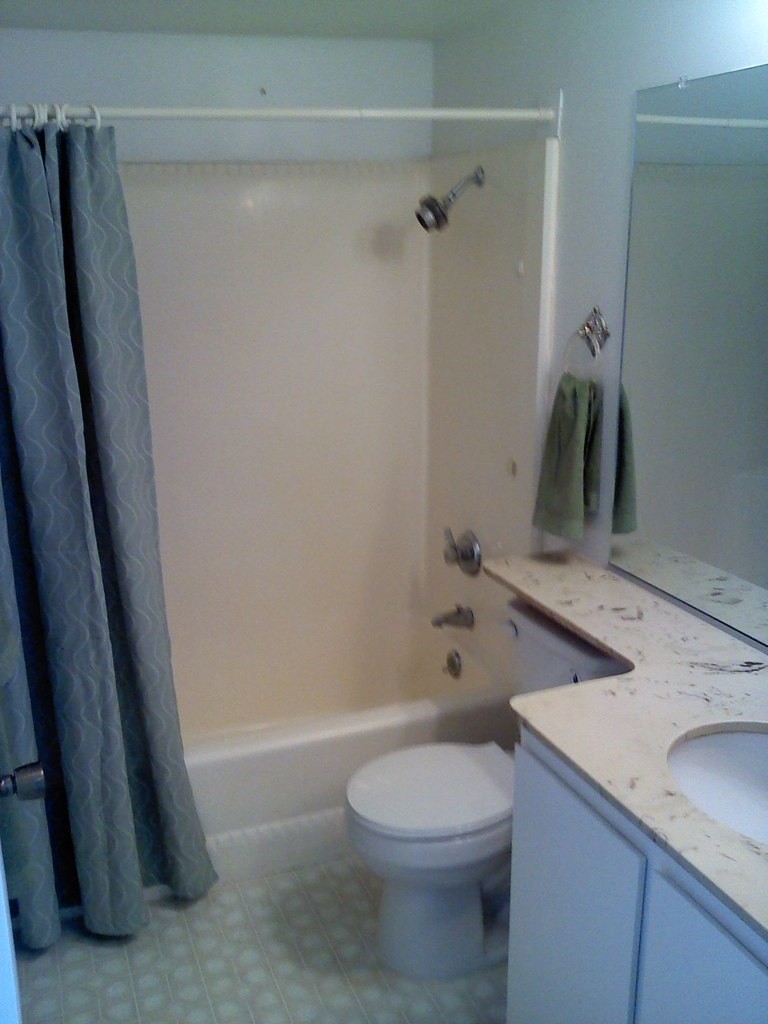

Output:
xmin=608 ymin=64 xmax=768 ymax=646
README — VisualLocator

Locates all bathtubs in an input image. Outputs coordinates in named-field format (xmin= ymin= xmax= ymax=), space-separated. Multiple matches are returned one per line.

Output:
xmin=176 ymin=601 xmax=577 ymax=876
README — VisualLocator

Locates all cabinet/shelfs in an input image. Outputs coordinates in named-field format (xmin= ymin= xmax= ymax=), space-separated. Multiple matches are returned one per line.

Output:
xmin=506 ymin=725 xmax=768 ymax=1024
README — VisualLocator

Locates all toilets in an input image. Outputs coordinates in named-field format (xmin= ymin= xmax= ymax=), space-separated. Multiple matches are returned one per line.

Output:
xmin=340 ymin=597 xmax=626 ymax=981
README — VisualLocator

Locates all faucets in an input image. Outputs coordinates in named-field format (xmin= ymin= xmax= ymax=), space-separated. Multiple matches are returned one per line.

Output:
xmin=429 ymin=601 xmax=475 ymax=631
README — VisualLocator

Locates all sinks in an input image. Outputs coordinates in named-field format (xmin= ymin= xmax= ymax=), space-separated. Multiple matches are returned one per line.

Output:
xmin=664 ymin=718 xmax=768 ymax=851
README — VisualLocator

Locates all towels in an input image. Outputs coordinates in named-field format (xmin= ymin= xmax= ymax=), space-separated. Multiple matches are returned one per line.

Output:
xmin=612 ymin=381 xmax=638 ymax=536
xmin=530 ymin=371 xmax=603 ymax=541
